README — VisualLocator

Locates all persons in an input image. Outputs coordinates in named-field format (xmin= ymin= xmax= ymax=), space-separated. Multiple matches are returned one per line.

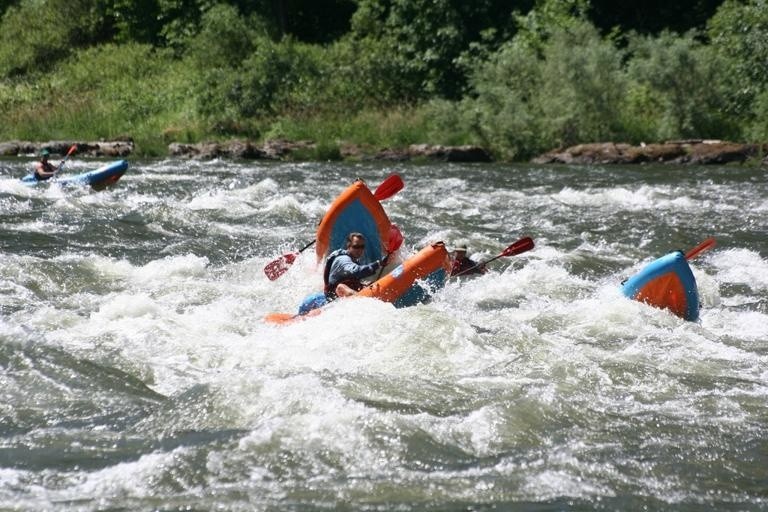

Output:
xmin=451 ymin=244 xmax=485 ymax=275
xmin=33 ymin=150 xmax=65 ymax=180
xmin=324 ymin=233 xmax=397 ymax=301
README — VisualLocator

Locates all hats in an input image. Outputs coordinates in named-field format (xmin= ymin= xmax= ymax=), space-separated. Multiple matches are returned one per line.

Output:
xmin=453 ymin=242 xmax=468 ymax=253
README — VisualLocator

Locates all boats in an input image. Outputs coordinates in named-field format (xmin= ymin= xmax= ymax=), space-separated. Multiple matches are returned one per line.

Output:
xmin=9 ymin=159 xmax=128 ymax=196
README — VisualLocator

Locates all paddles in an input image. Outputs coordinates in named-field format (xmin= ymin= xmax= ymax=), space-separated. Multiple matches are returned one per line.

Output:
xmin=55 ymin=144 xmax=78 ymax=176
xmin=685 ymin=239 xmax=715 ymax=260
xmin=376 ymin=224 xmax=402 ymax=279
xmin=454 ymin=237 xmax=535 ymax=277
xmin=265 ymin=175 xmax=405 ymax=281
